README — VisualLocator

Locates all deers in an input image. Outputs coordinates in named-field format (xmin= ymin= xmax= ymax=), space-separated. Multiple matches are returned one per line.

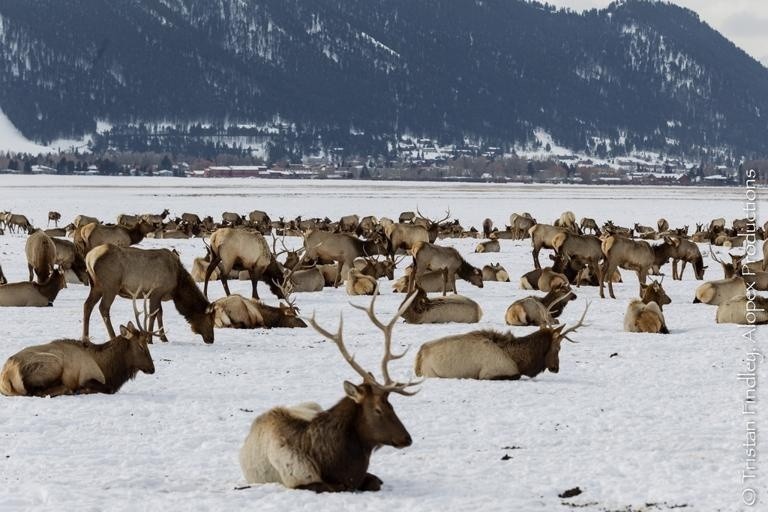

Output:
xmin=1 ymin=206 xmax=763 ymax=494
